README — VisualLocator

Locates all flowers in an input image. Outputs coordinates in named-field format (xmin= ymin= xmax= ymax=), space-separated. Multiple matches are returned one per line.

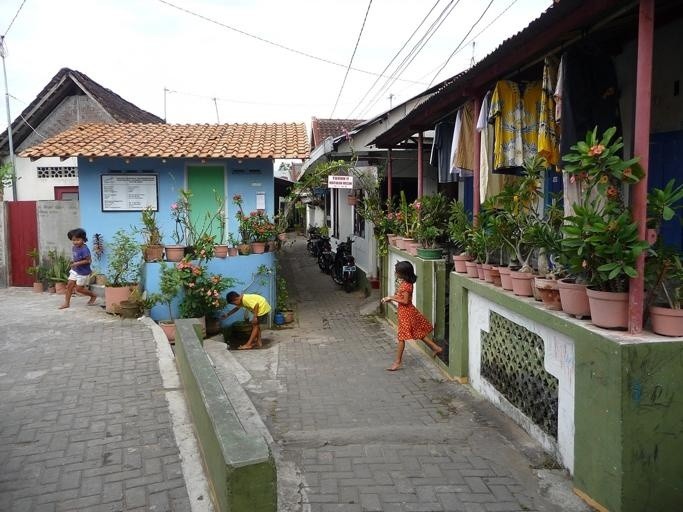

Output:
xmin=139 ymin=183 xmax=280 ymax=264
xmin=177 ymin=259 xmax=236 ymax=317
xmin=383 ymin=122 xmax=682 ymax=340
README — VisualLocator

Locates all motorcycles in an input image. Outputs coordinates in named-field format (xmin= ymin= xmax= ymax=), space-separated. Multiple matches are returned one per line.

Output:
xmin=307 ymin=225 xmax=334 ymax=275
xmin=331 ymin=236 xmax=360 ymax=293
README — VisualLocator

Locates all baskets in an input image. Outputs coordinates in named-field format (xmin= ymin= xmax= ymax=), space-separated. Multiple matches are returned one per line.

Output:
xmin=347 ymin=194 xmax=355 ymax=205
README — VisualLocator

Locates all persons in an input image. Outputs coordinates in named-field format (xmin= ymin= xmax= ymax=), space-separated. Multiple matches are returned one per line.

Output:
xmin=379 ymin=260 xmax=442 ymax=372
xmin=220 ymin=290 xmax=272 ymax=351
xmin=57 ymin=227 xmax=97 ymax=309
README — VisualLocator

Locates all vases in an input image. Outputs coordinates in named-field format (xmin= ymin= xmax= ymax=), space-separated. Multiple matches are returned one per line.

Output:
xmin=189 ymin=315 xmax=206 ymax=340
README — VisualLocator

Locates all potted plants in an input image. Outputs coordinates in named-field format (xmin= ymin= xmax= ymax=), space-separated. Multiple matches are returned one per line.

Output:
xmin=23 ymin=226 xmax=151 ymax=321
xmin=275 ymin=276 xmax=293 ymax=323
xmin=153 ymin=263 xmax=178 ymax=347
xmin=274 ymin=294 xmax=285 ymax=325
